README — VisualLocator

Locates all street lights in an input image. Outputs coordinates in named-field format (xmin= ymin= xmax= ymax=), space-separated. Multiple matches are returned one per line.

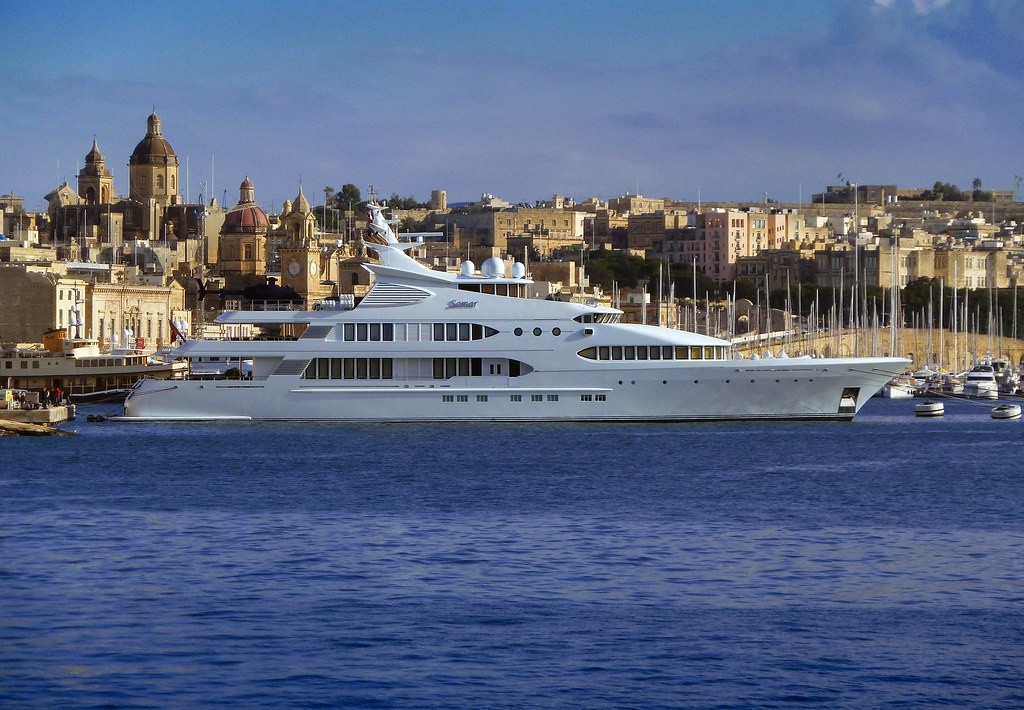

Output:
xmin=590 ymin=219 xmax=595 ymax=250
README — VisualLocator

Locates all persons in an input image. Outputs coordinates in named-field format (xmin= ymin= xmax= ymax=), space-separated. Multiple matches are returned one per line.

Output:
xmin=16 ymin=387 xmax=72 ymax=410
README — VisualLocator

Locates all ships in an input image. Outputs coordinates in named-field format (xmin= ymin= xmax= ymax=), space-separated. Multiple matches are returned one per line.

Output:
xmin=106 ymin=204 xmax=912 ymax=422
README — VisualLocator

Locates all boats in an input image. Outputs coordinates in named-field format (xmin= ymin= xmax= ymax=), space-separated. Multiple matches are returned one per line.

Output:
xmin=873 ymin=353 xmax=1024 ymax=419
xmin=0 ymin=329 xmax=191 ymax=401
